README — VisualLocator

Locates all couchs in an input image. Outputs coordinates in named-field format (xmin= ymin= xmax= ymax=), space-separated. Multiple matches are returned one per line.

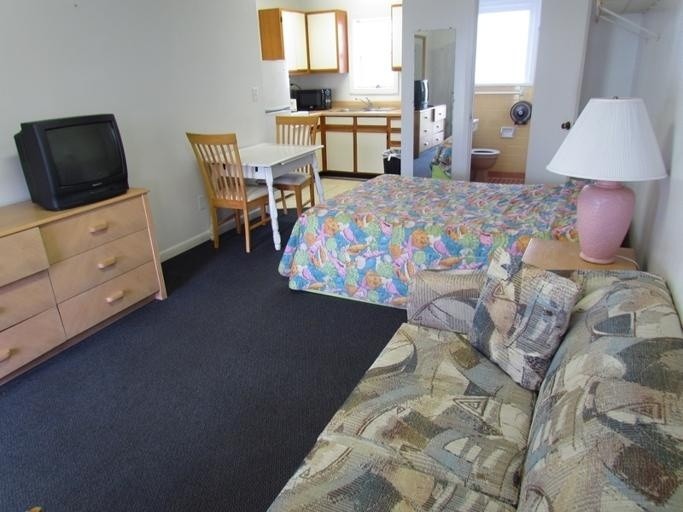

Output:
xmin=263 ymin=269 xmax=681 ymax=511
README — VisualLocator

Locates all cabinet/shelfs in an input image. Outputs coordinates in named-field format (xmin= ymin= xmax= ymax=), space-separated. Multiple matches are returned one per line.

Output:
xmin=0 ymin=188 xmax=168 ymax=390
xmin=414 ymin=104 xmax=447 ymax=158
xmin=309 ymin=116 xmax=324 ymax=174
xmin=388 ymin=115 xmax=401 ymax=148
xmin=322 ymin=117 xmax=390 ymax=180
xmin=306 ymin=10 xmax=349 ymax=75
xmin=258 ymin=8 xmax=310 ymax=77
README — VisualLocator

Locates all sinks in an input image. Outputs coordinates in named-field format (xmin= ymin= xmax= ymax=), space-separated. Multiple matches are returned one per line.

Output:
xmin=369 ymin=109 xmax=392 ymax=112
xmin=334 ymin=109 xmax=356 ymax=112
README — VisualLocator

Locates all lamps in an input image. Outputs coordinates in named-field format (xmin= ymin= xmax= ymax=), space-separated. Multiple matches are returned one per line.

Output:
xmin=547 ymin=98 xmax=668 ymax=265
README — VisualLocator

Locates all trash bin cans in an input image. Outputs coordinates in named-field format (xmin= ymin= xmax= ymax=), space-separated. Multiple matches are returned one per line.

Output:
xmin=382 ymin=149 xmax=400 ymax=174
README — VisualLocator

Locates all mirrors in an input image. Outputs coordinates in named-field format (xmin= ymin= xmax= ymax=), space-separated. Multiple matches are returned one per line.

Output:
xmin=414 ymin=28 xmax=456 ymax=180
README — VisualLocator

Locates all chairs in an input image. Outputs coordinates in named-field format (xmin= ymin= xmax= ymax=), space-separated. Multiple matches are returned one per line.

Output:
xmin=186 ymin=132 xmax=277 ymax=253
xmin=256 ymin=115 xmax=322 ymax=225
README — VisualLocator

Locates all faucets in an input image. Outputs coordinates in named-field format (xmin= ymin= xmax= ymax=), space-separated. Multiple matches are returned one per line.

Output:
xmin=354 ymin=98 xmax=375 ymax=110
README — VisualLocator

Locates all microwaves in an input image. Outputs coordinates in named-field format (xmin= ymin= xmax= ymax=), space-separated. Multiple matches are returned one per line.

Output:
xmin=291 ymin=89 xmax=332 ymax=110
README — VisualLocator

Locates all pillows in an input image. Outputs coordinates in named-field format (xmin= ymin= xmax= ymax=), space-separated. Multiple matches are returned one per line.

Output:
xmin=467 ymin=246 xmax=580 ymax=392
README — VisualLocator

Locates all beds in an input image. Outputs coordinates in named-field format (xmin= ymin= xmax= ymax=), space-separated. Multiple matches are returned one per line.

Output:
xmin=414 ymin=136 xmax=452 ymax=179
xmin=278 ymin=174 xmax=587 ymax=309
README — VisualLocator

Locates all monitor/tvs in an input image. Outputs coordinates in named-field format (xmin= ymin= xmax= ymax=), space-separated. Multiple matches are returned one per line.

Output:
xmin=414 ymin=79 xmax=429 ymax=111
xmin=14 ymin=113 xmax=128 ymax=211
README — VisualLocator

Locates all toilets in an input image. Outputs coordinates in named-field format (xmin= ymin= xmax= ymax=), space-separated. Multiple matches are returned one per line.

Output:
xmin=471 ymin=148 xmax=501 ymax=182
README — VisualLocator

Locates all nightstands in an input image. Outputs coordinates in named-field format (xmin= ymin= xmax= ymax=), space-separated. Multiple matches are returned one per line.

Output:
xmin=521 ymin=236 xmax=638 ymax=279
